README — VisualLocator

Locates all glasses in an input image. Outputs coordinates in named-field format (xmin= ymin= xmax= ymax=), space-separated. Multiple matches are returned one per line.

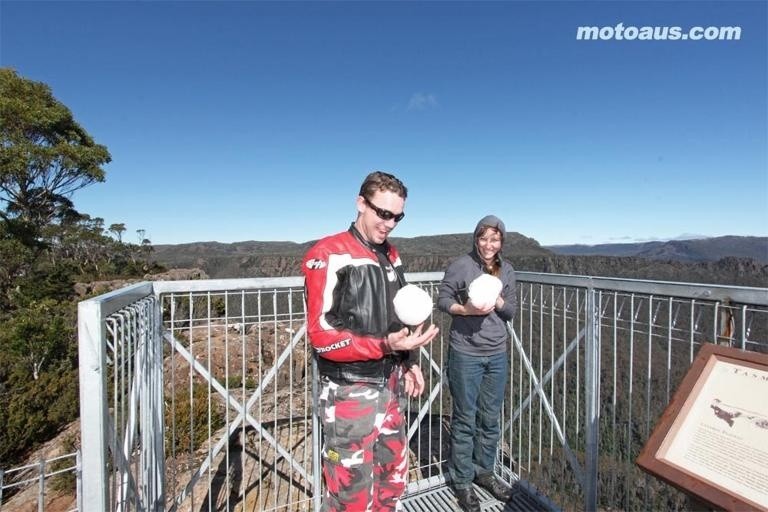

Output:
xmin=364 ymin=196 xmax=404 ymax=222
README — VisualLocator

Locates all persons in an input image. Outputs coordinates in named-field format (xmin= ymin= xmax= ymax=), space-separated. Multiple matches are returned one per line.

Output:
xmin=434 ymin=213 xmax=519 ymax=511
xmin=298 ymin=169 xmax=440 ymax=511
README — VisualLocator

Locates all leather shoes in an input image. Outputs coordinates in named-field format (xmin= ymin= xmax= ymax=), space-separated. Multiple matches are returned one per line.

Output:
xmin=481 ymin=474 xmax=512 ymax=502
xmin=456 ymin=488 xmax=479 ymax=512
